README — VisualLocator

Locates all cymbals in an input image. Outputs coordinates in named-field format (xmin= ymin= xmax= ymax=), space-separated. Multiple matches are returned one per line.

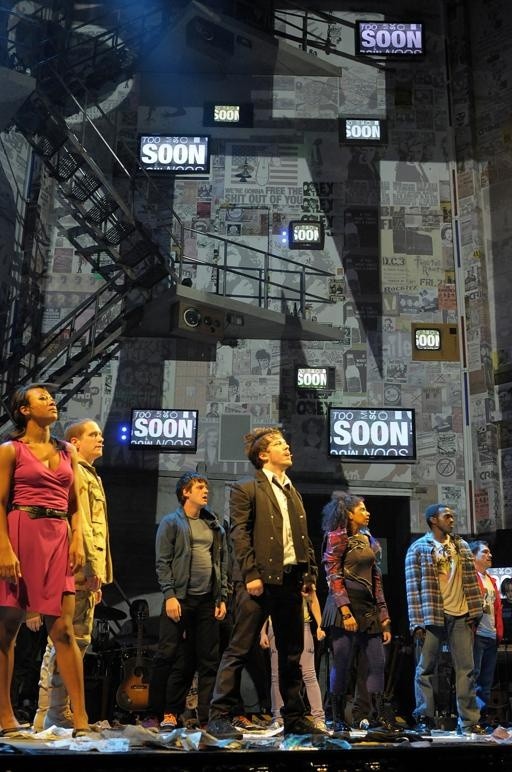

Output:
xmin=94 ymin=606 xmax=127 ymax=620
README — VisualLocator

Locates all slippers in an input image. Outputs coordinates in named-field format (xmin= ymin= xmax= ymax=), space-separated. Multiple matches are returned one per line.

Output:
xmin=0 ymin=726 xmax=32 ymax=739
xmin=72 ymin=728 xmax=92 ymax=738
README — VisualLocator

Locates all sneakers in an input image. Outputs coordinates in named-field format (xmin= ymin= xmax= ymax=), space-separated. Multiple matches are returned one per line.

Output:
xmin=194 ymin=712 xmax=274 ymax=740
xmin=142 ymin=717 xmax=161 ymax=734
xmin=415 ymin=713 xmax=435 ymax=735
xmin=455 ymin=722 xmax=488 ymax=737
xmin=282 ymin=712 xmax=333 ymax=741
xmin=160 ymin=713 xmax=178 ymax=732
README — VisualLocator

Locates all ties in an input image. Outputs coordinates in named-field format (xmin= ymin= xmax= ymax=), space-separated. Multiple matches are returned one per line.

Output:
xmin=271 ymin=476 xmax=308 ymax=563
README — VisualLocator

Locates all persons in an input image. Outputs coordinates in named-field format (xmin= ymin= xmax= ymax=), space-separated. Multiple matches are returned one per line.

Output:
xmin=226 ymin=376 xmax=240 ymax=402
xmin=316 ymin=492 xmax=401 ymax=732
xmin=156 ymin=426 xmax=326 ymax=739
xmin=441 ymin=225 xmax=453 ymax=248
xmin=251 ymin=349 xmax=271 ymax=375
xmin=347 ymin=146 xmax=379 ymax=179
xmin=396 ymin=137 xmax=430 ymax=182
xmin=1 ymin=385 xmax=114 ymax=738
xmin=405 ymin=504 xmax=512 ymax=735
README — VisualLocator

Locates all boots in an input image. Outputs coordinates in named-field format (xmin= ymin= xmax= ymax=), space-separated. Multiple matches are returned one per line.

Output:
xmin=31 ymin=635 xmax=103 ymax=734
xmin=368 ymin=691 xmax=405 ymax=734
xmin=329 ymin=691 xmax=352 ymax=736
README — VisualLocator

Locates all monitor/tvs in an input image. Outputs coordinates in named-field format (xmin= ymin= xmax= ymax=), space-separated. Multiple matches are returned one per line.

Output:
xmin=485 ymin=566 xmax=512 ymax=601
xmin=339 ymin=118 xmax=388 ymax=145
xmin=414 ymin=328 xmax=441 ymax=351
xmin=296 ymin=366 xmax=334 ymax=389
xmin=328 ymin=407 xmax=415 ymax=459
xmin=137 ymin=132 xmax=211 ymax=180
xmin=129 ymin=408 xmax=198 ymax=454
xmin=289 ymin=220 xmax=324 ymax=250
xmin=212 ymin=104 xmax=241 ymax=124
xmin=356 ymin=20 xmax=425 ymax=56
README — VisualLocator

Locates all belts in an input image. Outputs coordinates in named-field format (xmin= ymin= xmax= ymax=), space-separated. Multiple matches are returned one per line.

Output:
xmin=10 ymin=504 xmax=68 ymax=520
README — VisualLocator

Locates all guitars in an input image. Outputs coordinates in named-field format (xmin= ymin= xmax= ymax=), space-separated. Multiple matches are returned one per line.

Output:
xmin=116 ymin=611 xmax=149 ymax=712
